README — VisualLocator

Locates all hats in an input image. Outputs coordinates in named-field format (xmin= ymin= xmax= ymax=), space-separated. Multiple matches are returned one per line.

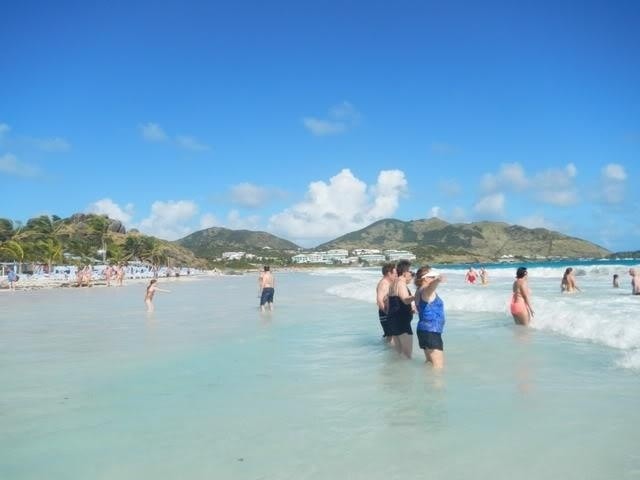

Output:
xmin=421 ymin=270 xmax=438 ymax=279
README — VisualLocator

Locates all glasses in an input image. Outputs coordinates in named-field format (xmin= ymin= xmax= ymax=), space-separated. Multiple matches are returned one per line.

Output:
xmin=404 ymin=269 xmax=415 ymax=277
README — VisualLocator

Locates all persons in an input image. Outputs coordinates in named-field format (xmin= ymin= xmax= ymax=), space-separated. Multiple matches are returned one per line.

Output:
xmin=6 ymin=267 xmax=17 ymax=291
xmin=629 ymin=267 xmax=640 ymax=296
xmin=479 ymin=266 xmax=488 ymax=285
xmin=510 ymin=267 xmax=535 ymax=326
xmin=561 ymin=267 xmax=583 ymax=294
xmin=145 ymin=280 xmax=171 ymax=312
xmin=414 ymin=264 xmax=445 ymax=371
xmin=376 ymin=260 xmax=416 ymax=359
xmin=260 ymin=266 xmax=274 ymax=312
xmin=465 ymin=266 xmax=478 ymax=284
xmin=613 ymin=274 xmax=619 ymax=287
xmin=67 ymin=263 xmax=124 ymax=287
